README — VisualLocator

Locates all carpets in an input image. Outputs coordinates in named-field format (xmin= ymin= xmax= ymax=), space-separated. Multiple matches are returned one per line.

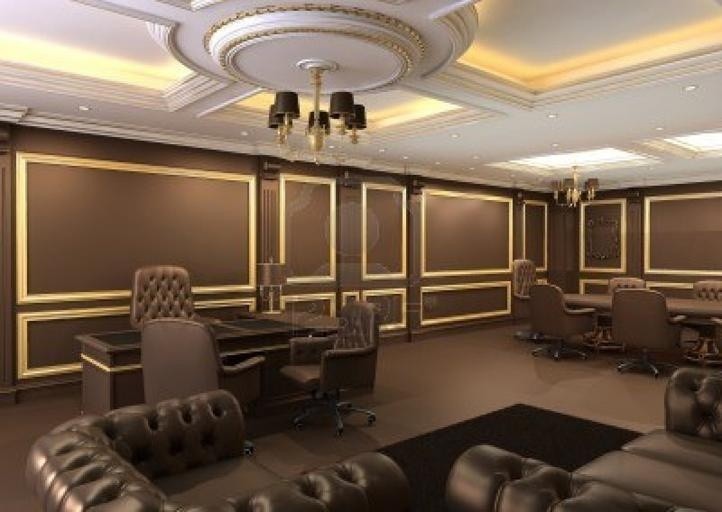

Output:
xmin=373 ymin=403 xmax=646 ymax=512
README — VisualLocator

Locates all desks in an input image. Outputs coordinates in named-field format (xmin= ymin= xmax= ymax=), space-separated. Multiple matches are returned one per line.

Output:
xmin=558 ymin=292 xmax=722 ymax=349
xmin=72 ymin=311 xmax=353 ymax=441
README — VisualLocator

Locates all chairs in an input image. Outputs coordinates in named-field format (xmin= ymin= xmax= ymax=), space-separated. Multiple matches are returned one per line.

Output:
xmin=441 ymin=366 xmax=722 ymax=512
xmin=683 ymin=279 xmax=722 ymax=362
xmin=512 ymin=259 xmax=597 ymax=361
xmin=24 ymin=389 xmax=414 ymax=512
xmin=605 ymin=276 xmax=648 ymax=296
xmin=611 ymin=286 xmax=687 ymax=378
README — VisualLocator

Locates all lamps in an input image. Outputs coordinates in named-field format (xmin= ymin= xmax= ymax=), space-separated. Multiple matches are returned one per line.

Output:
xmin=267 ymin=57 xmax=367 ymax=166
xmin=256 ymin=256 xmax=288 ymax=315
xmin=549 ymin=163 xmax=599 ymax=208
